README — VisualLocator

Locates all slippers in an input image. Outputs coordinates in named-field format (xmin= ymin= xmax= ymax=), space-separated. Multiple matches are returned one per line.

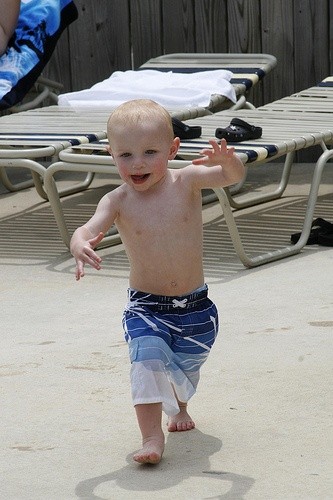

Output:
xmin=215 ymin=118 xmax=262 ymax=142
xmin=291 ymin=218 xmax=333 ymax=247
xmin=171 ymin=116 xmax=202 ymax=139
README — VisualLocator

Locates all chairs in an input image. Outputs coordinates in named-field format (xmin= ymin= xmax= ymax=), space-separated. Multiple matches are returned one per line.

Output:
xmin=0 ymin=54 xmax=279 ymax=201
xmin=0 ymin=0 xmax=78 ymax=192
xmin=46 ymin=77 xmax=333 ymax=268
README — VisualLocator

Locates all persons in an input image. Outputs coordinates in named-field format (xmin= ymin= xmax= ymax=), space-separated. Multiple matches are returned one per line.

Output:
xmin=0 ymin=0 xmax=21 ymax=58
xmin=70 ymin=99 xmax=247 ymax=464
